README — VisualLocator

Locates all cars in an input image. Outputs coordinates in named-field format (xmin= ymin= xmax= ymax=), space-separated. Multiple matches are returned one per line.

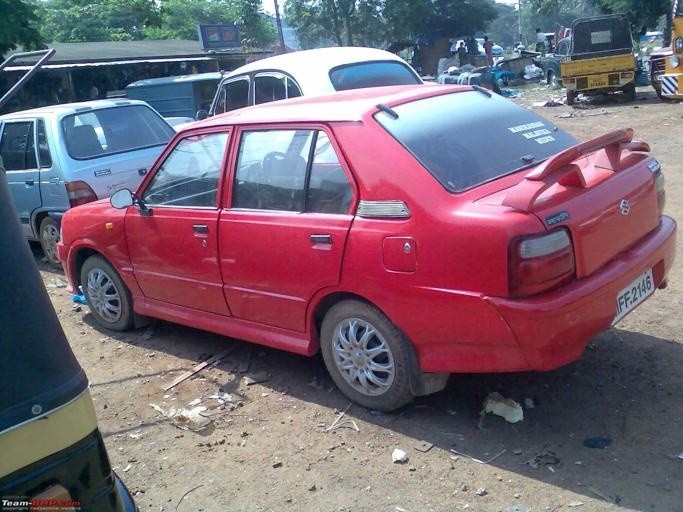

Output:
xmin=540 ymin=37 xmax=572 ymax=89
xmin=57 ymin=84 xmax=677 ymax=414
xmin=0 ymin=97 xmax=201 ymax=270
xmin=0 ymin=154 xmax=139 ymax=512
xmin=172 ymin=46 xmax=426 ymax=172
xmin=456 ymin=38 xmax=503 ymax=56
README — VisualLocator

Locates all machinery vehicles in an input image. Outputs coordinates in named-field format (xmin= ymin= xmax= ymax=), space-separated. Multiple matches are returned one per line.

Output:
xmin=649 ymin=0 xmax=683 ymax=100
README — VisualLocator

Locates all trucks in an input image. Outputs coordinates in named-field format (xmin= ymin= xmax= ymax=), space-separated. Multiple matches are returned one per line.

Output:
xmin=559 ymin=13 xmax=640 ymax=105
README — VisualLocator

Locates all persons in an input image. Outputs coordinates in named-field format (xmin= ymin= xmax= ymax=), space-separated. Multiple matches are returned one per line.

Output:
xmin=534 ymin=26 xmax=547 ymax=57
xmin=449 ymin=41 xmax=453 ymax=57
xmin=483 ymin=35 xmax=495 ymax=68
xmin=458 ymin=41 xmax=467 ymax=66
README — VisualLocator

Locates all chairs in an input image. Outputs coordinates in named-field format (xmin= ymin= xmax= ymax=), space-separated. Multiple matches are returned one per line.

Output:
xmin=68 ymin=125 xmax=104 ymax=158
